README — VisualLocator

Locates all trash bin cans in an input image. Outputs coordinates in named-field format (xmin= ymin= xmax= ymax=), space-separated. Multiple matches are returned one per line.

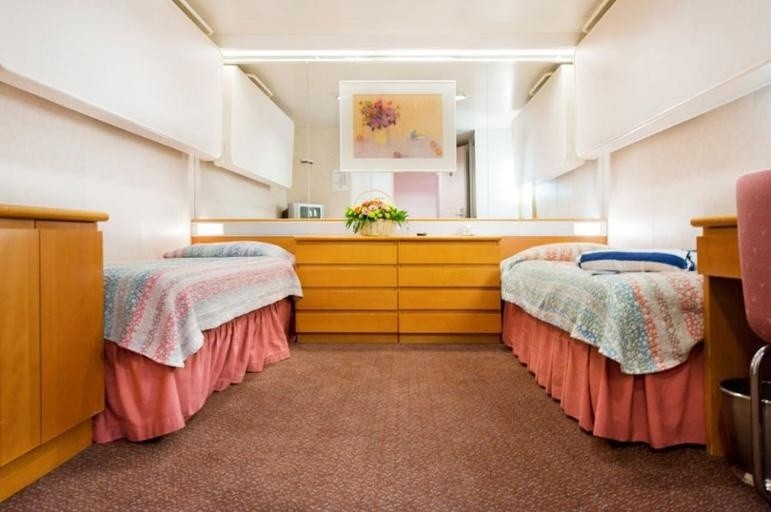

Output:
xmin=716 ymin=344 xmax=771 ymax=503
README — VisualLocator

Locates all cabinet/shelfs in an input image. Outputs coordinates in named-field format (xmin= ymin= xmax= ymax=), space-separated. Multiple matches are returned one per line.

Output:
xmin=293 ymin=237 xmax=503 ymax=346
xmin=0 ymin=203 xmax=110 ymax=505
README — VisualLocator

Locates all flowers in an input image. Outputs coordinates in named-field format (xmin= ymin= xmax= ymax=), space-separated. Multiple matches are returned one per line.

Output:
xmin=345 ymin=200 xmax=411 ymax=235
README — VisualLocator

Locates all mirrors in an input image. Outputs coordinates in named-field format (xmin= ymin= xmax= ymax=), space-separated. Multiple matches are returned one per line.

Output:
xmin=189 ymin=57 xmax=610 ymax=224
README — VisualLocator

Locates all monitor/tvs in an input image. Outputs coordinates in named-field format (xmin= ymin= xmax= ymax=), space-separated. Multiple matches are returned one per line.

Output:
xmin=281 ymin=202 xmax=325 ymax=218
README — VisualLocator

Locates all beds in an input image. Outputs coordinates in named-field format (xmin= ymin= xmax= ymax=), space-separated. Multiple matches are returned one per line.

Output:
xmin=499 ymin=236 xmax=707 ymax=450
xmin=91 ymin=235 xmax=304 ymax=446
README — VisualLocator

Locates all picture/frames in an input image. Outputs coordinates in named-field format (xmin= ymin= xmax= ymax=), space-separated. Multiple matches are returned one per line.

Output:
xmin=338 ymin=79 xmax=458 ymax=173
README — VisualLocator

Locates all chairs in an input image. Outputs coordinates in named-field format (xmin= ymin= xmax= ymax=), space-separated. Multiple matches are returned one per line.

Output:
xmin=735 ymin=170 xmax=770 ymax=505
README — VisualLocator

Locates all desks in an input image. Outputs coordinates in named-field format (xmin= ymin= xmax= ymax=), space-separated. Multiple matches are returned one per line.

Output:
xmin=689 ymin=213 xmax=742 ymax=459
